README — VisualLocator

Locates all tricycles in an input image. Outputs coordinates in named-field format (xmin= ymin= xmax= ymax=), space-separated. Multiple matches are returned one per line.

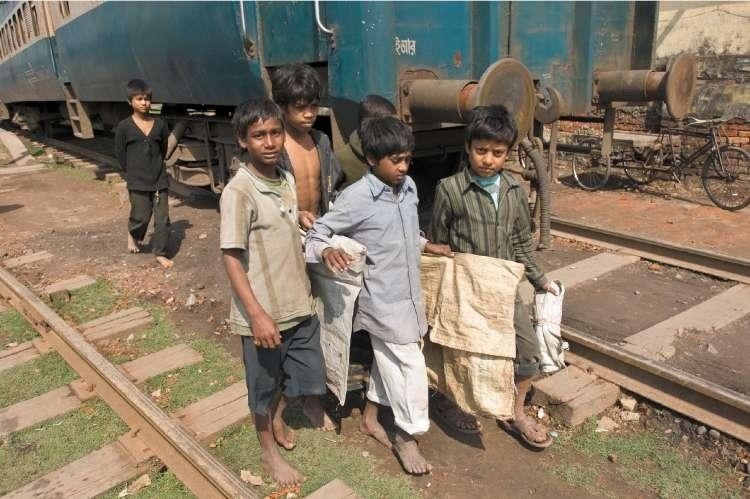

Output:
xmin=571 ymin=116 xmax=750 ymax=211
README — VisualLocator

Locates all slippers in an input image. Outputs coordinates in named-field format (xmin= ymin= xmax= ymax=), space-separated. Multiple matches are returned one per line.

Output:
xmin=499 ymin=414 xmax=554 ymax=449
xmin=433 ymin=398 xmax=483 ymax=435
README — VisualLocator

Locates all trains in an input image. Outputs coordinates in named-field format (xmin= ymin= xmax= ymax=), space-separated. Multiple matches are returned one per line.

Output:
xmin=1 ymin=1 xmax=701 ymax=252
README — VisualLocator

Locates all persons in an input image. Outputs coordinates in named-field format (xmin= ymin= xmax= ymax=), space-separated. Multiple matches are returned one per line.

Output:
xmin=334 ymin=95 xmax=396 ymax=192
xmin=305 ymin=117 xmax=454 ymax=474
xmin=427 ymin=105 xmax=557 ymax=448
xmin=271 ymin=63 xmax=341 ymax=230
xmin=115 ymin=78 xmax=174 ymax=268
xmin=221 ymin=97 xmax=327 ymax=489
